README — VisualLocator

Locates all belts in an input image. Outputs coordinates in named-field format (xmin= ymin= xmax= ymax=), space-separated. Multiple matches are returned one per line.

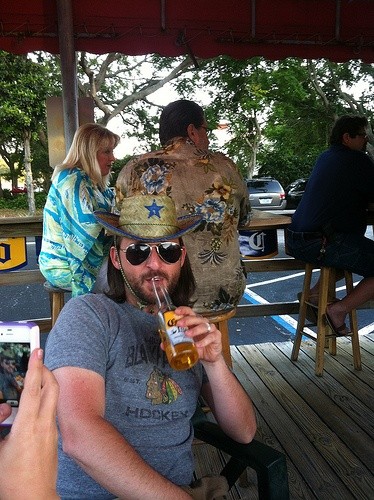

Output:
xmin=285 ymin=229 xmax=325 ymax=239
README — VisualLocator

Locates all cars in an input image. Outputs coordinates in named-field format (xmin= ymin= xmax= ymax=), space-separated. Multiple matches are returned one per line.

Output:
xmin=284 ymin=178 xmax=308 ymax=210
xmin=9 ymin=186 xmax=27 ymax=196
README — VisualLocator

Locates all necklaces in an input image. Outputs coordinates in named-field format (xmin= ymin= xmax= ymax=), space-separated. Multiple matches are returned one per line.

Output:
xmin=147 ymin=307 xmax=155 ymax=314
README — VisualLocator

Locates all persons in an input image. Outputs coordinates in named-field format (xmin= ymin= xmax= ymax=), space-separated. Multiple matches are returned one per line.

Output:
xmin=1 ymin=349 xmax=26 ymax=406
xmin=37 ymin=122 xmax=119 ymax=298
xmin=113 ymin=99 xmax=251 ymax=315
xmin=43 ymin=195 xmax=258 ymax=500
xmin=284 ymin=115 xmax=374 ymax=338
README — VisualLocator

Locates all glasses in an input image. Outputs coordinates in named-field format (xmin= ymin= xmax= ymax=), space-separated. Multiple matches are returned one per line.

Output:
xmin=4 ymin=360 xmax=16 ymax=365
xmin=120 ymin=241 xmax=184 ymax=267
xmin=202 ymin=126 xmax=212 ymax=137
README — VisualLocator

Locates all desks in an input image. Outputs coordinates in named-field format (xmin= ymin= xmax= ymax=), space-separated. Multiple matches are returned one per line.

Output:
xmin=0 ymin=208 xmax=374 ymax=330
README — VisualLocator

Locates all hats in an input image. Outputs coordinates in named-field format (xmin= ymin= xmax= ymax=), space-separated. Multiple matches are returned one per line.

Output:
xmin=0 ymin=349 xmax=16 ymax=359
xmin=94 ymin=195 xmax=203 ymax=242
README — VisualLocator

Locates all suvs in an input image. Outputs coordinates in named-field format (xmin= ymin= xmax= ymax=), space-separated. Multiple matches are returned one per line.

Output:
xmin=243 ymin=177 xmax=287 ymax=211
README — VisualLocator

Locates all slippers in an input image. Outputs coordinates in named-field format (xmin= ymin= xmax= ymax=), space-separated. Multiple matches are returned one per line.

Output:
xmin=297 ymin=292 xmax=341 ymax=308
xmin=325 ymin=303 xmax=346 ymax=337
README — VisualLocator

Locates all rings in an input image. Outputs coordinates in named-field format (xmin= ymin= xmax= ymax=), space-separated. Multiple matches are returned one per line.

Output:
xmin=203 ymin=322 xmax=215 ymax=335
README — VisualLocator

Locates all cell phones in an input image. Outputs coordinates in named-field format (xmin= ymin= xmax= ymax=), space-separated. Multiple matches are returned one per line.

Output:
xmin=0 ymin=320 xmax=41 ymax=428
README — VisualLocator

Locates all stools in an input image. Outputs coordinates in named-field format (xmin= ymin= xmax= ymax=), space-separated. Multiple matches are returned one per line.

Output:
xmin=195 ymin=308 xmax=236 ymax=372
xmin=42 ymin=281 xmax=74 ymax=330
xmin=292 ymin=262 xmax=362 ymax=378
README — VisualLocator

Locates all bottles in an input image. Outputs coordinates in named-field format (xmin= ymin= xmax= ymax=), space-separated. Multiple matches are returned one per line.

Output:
xmin=10 ymin=369 xmax=25 ymax=393
xmin=151 ymin=276 xmax=199 ymax=371
